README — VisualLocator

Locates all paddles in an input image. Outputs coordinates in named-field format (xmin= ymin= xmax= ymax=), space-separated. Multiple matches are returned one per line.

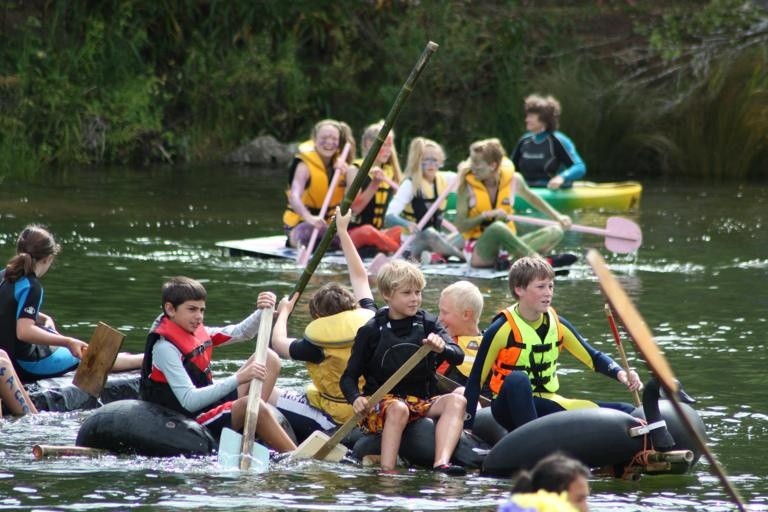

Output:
xmin=216 ymin=292 xmax=278 ymax=476
xmin=366 ymin=165 xmax=465 ymax=288
xmin=507 ymin=215 xmax=641 ymax=255
xmin=291 ymin=344 xmax=433 ymax=463
xmin=583 ymin=247 xmax=748 ymax=512
xmin=34 ymin=321 xmax=126 ymax=399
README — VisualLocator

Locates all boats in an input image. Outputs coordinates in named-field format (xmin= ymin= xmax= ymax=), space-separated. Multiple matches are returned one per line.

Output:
xmin=212 ymin=223 xmax=581 ymax=280
xmin=0 ymin=361 xmax=144 ymax=416
xmin=72 ymin=378 xmax=707 ymax=483
xmin=438 ymin=169 xmax=642 ymax=223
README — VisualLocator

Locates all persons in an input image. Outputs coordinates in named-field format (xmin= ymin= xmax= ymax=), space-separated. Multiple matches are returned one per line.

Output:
xmin=491 ymin=450 xmax=592 ymax=512
xmin=346 ymin=118 xmax=405 ymax=259
xmin=285 ymin=118 xmax=356 ymax=255
xmin=433 ymin=279 xmax=494 ymax=408
xmin=339 ymin=258 xmax=467 ymax=484
xmin=138 ymin=275 xmax=298 ymax=453
xmin=453 ymin=136 xmax=574 ymax=267
xmin=464 ymin=254 xmax=644 ymax=433
xmin=508 ymin=91 xmax=589 ymax=191
xmin=0 ymin=349 xmax=39 ymax=417
xmin=0 ymin=221 xmax=144 ymax=385
xmin=382 ymin=135 xmax=467 ymax=266
xmin=271 ymin=206 xmax=380 ymax=431
